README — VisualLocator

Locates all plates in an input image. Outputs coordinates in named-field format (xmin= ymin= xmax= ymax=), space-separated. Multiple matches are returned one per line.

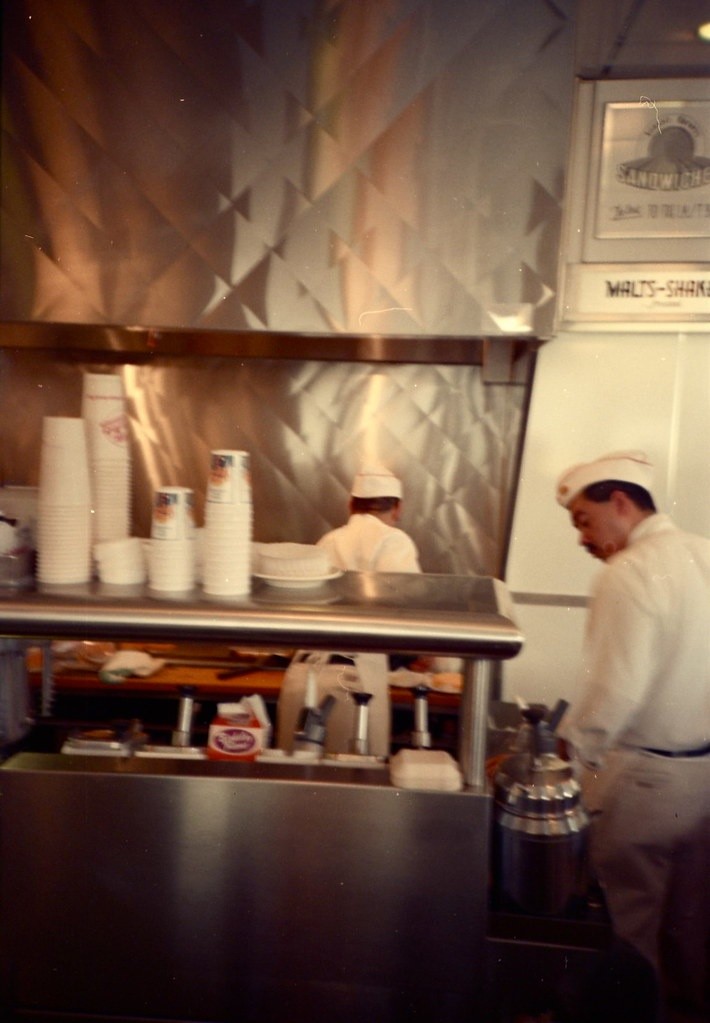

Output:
xmin=252 ymin=569 xmax=343 ymax=588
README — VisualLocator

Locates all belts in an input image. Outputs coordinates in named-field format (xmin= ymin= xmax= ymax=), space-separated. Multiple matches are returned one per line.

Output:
xmin=643 ymin=744 xmax=710 ymax=757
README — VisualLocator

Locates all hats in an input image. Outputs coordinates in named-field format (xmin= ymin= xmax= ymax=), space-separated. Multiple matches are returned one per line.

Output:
xmin=556 ymin=451 xmax=658 ymax=506
xmin=349 ymin=462 xmax=400 ymax=498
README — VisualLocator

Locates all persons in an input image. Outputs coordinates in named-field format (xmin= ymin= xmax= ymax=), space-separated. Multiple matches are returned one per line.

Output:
xmin=313 ymin=467 xmax=421 ymax=574
xmin=554 ymin=448 xmax=710 ymax=1023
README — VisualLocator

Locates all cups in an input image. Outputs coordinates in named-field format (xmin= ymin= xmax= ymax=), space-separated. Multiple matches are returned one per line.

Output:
xmin=35 ymin=372 xmax=254 ymax=599
